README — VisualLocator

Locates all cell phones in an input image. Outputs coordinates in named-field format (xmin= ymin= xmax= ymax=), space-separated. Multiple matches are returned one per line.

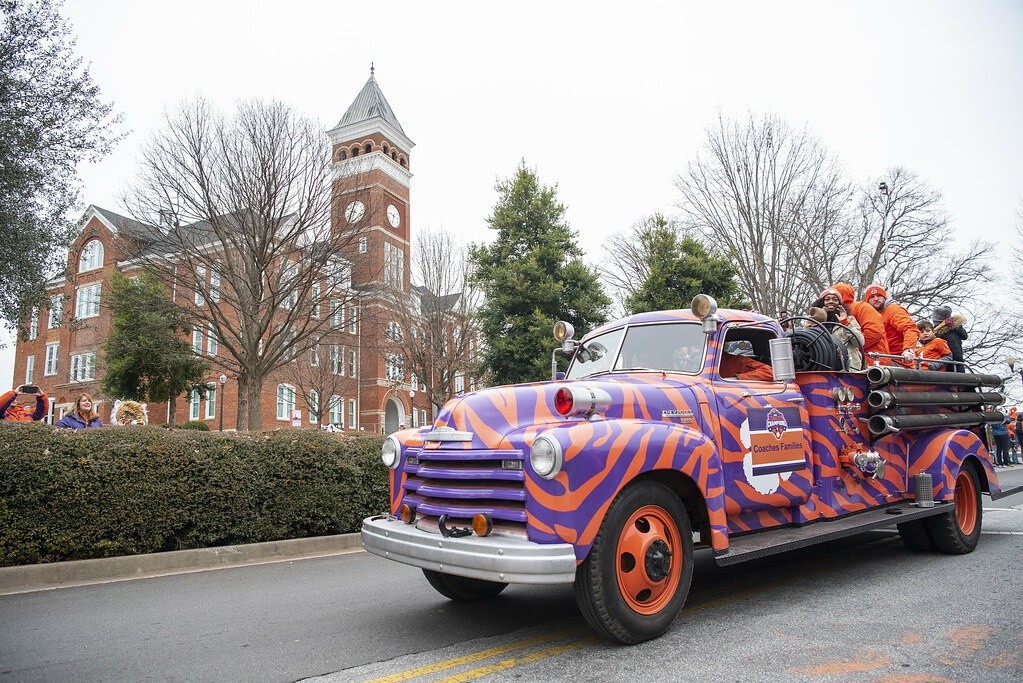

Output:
xmin=22 ymin=386 xmax=37 ymax=393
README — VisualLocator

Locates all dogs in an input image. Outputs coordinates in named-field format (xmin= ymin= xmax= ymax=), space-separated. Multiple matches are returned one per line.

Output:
xmin=804 ymin=306 xmax=828 ymax=331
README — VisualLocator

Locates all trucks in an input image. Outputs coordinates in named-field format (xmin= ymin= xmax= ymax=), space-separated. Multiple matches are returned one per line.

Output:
xmin=358 ymin=294 xmax=1004 ymax=648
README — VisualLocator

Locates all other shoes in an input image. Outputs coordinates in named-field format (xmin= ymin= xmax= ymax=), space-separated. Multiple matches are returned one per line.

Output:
xmin=998 ymin=463 xmax=1003 ymax=467
xmin=1003 ymin=461 xmax=1015 ymax=467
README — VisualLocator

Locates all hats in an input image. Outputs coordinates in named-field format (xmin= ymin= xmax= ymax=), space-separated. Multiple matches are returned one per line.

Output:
xmin=931 ymin=306 xmax=952 ymax=325
xmin=865 ymin=286 xmax=887 ymax=303
xmin=832 ymin=283 xmax=855 ymax=303
xmin=1005 ymin=416 xmax=1010 ymax=423
xmin=818 ymin=287 xmax=842 ymax=304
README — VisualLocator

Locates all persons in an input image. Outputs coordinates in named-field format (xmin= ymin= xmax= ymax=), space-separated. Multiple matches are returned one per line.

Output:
xmin=915 ymin=320 xmax=952 ymax=371
xmin=932 ymin=305 xmax=969 ymax=373
xmin=990 ymin=406 xmax=1023 ymax=468
xmin=719 ymin=339 xmax=774 ymax=381
xmin=828 ymin=284 xmax=892 ymax=368
xmin=863 ymin=284 xmax=921 ymax=370
xmin=804 ymin=288 xmax=865 ymax=372
xmin=778 ymin=310 xmax=792 ymax=332
xmin=54 ymin=393 xmax=104 ymax=429
xmin=0 ymin=383 xmax=50 ymax=423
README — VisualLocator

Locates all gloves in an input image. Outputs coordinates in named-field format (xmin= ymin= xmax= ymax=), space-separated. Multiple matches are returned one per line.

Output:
xmin=901 ymin=348 xmax=914 ymax=363
xmin=927 ymin=362 xmax=937 ymax=371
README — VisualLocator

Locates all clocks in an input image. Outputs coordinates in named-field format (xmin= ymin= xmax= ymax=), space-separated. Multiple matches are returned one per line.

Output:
xmin=345 ymin=199 xmax=365 ymax=225
xmin=387 ymin=204 xmax=401 ymax=230
